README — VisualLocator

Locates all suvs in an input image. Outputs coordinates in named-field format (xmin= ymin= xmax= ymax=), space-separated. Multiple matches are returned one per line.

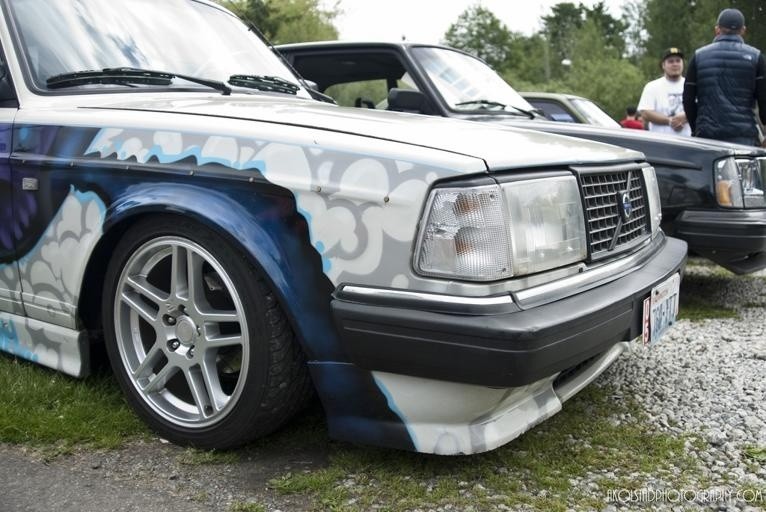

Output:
xmin=263 ymin=37 xmax=763 ymax=280
xmin=0 ymin=1 xmax=692 ymax=469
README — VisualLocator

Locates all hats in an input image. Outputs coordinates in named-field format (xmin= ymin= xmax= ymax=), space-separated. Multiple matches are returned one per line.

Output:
xmin=660 ymin=47 xmax=686 ymax=60
xmin=714 ymin=8 xmax=745 ymax=31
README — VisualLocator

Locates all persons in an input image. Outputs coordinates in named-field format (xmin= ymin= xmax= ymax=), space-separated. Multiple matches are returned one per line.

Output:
xmin=636 ymin=47 xmax=691 ymax=137
xmin=620 ymin=106 xmax=644 ymax=130
xmin=682 ymin=8 xmax=766 ymax=147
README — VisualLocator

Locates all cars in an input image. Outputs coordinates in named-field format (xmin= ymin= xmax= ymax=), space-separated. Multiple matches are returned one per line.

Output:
xmin=520 ymin=90 xmax=627 ymax=130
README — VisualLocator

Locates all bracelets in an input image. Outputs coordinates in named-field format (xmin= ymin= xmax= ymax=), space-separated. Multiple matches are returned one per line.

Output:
xmin=668 ymin=116 xmax=673 ymax=127
